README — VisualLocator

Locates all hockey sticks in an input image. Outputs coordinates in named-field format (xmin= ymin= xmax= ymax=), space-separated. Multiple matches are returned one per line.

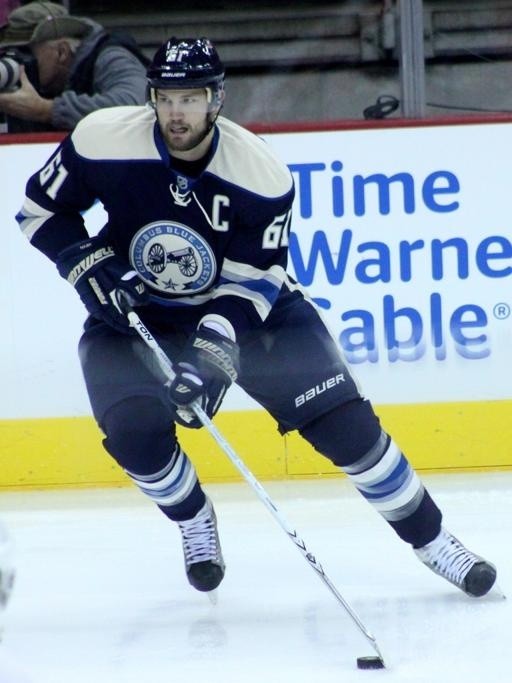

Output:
xmin=121 ymin=294 xmax=390 ymax=667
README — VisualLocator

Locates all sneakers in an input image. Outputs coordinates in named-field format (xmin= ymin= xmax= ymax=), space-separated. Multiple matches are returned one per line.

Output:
xmin=419 ymin=527 xmax=495 ymax=595
xmin=176 ymin=492 xmax=225 ymax=593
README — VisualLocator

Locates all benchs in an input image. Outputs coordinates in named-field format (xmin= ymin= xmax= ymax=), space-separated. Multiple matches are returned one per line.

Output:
xmin=92 ymin=3 xmax=512 ymax=70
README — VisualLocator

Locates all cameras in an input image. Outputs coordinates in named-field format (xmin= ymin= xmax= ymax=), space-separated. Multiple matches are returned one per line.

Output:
xmin=0 ymin=41 xmax=40 ymax=98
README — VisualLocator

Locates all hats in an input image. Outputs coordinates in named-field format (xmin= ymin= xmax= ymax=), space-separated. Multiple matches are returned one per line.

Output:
xmin=1 ymin=0 xmax=91 ymax=49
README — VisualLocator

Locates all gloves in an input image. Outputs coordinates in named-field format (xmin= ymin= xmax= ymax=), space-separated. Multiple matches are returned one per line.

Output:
xmin=55 ymin=236 xmax=148 ymax=333
xmin=160 ymin=322 xmax=242 ymax=431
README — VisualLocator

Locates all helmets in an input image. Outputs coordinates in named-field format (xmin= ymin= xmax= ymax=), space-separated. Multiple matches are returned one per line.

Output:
xmin=143 ymin=35 xmax=226 ymax=108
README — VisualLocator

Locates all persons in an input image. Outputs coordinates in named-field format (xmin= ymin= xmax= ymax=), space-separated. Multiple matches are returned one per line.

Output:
xmin=0 ymin=0 xmax=154 ymax=132
xmin=12 ymin=36 xmax=499 ymax=598
